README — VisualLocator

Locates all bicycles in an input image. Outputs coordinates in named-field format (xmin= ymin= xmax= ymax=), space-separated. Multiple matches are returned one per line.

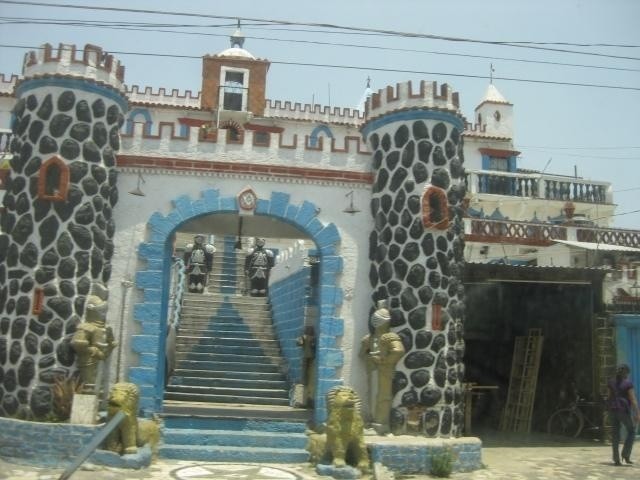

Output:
xmin=546 ymin=388 xmax=613 ymax=440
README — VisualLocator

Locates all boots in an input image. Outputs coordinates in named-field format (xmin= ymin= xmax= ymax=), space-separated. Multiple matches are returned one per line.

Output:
xmin=190 ymin=282 xmax=203 ymax=292
xmin=251 ymin=288 xmax=265 ymax=296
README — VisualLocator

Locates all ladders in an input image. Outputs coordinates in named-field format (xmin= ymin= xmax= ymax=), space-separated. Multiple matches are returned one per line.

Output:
xmin=502 ymin=327 xmax=544 ymax=434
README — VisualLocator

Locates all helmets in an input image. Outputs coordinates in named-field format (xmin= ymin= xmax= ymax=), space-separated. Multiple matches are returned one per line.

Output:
xmin=255 ymin=237 xmax=265 ymax=244
xmin=193 ymin=234 xmax=204 ymax=239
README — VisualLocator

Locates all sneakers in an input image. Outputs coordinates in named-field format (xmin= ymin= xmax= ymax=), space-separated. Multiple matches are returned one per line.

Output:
xmin=615 ymin=460 xmax=621 ymax=465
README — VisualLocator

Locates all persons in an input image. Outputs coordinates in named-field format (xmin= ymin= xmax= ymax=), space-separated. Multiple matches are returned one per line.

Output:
xmin=244 ymin=236 xmax=275 ymax=296
xmin=358 ymin=307 xmax=405 ymax=437
xmin=183 ymin=233 xmax=217 ymax=292
xmin=606 ymin=363 xmax=639 ymax=466
xmin=70 ymin=292 xmax=118 ymax=403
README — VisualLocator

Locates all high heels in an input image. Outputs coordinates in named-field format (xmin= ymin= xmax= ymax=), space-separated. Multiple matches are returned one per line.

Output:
xmin=621 ymin=451 xmax=632 ymax=464
